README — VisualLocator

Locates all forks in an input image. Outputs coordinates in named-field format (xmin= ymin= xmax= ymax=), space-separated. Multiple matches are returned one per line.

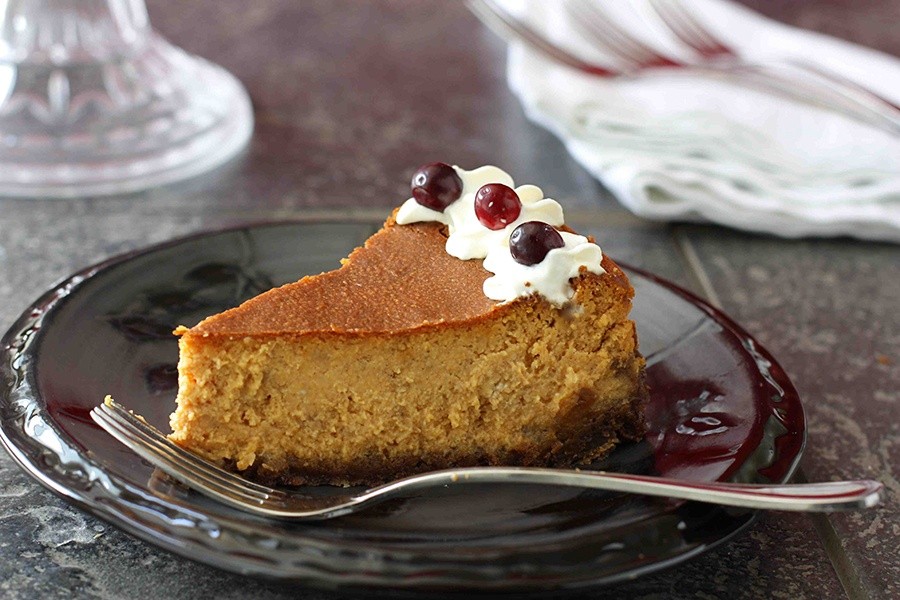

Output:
xmin=461 ymin=0 xmax=900 ymax=144
xmin=90 ymin=395 xmax=883 ymax=524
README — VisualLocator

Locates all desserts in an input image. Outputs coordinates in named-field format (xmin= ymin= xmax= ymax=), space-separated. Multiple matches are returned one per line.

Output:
xmin=165 ymin=162 xmax=644 ymax=488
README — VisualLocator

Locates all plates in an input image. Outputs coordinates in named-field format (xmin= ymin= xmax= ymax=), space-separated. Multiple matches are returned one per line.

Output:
xmin=0 ymin=211 xmax=806 ymax=598
